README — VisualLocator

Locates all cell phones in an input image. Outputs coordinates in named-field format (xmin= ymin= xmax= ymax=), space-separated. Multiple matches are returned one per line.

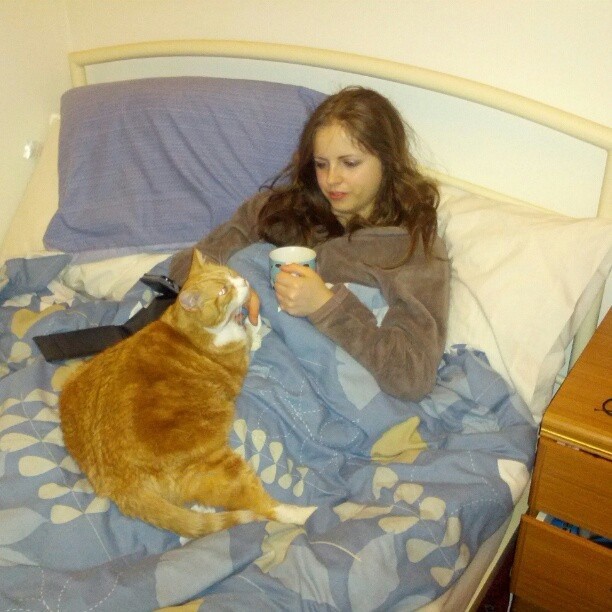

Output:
xmin=140 ymin=269 xmax=181 ymax=298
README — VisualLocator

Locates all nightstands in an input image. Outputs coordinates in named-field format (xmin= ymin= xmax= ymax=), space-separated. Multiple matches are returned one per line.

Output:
xmin=511 ymin=305 xmax=612 ymax=611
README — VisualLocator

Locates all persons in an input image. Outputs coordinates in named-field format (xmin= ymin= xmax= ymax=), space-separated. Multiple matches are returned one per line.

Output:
xmin=167 ymin=87 xmax=452 ymax=401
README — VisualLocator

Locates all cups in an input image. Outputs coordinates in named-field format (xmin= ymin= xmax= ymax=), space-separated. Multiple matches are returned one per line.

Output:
xmin=268 ymin=245 xmax=317 ymax=290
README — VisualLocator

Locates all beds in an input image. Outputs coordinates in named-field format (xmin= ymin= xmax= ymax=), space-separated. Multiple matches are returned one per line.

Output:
xmin=1 ymin=40 xmax=612 ymax=612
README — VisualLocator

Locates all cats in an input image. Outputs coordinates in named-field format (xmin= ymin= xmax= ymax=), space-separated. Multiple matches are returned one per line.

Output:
xmin=57 ymin=245 xmax=318 ymax=540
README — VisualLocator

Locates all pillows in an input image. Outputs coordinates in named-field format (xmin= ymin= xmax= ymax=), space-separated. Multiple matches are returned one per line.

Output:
xmin=44 ymin=76 xmax=333 ymax=254
